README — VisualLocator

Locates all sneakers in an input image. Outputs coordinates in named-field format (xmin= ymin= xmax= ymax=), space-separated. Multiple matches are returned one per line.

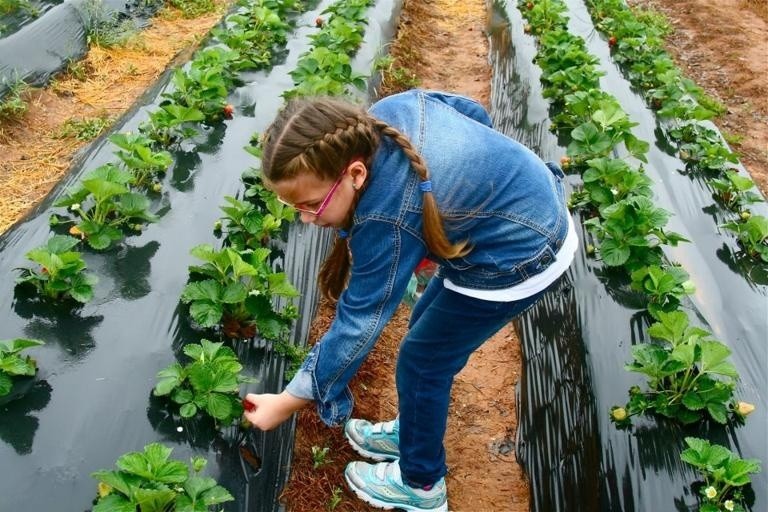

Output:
xmin=344 ymin=413 xmax=448 ymax=512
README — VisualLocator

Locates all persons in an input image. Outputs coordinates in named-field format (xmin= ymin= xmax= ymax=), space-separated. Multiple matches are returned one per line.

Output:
xmin=242 ymin=84 xmax=579 ymax=511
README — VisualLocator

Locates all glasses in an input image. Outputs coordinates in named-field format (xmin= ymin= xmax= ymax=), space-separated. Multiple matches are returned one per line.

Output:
xmin=277 ymin=160 xmax=356 ymax=216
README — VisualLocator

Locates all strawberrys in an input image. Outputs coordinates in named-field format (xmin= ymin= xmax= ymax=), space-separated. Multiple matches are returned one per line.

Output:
xmin=242 ymin=399 xmax=255 ymax=413
xmin=735 ymin=401 xmax=755 ymax=415
